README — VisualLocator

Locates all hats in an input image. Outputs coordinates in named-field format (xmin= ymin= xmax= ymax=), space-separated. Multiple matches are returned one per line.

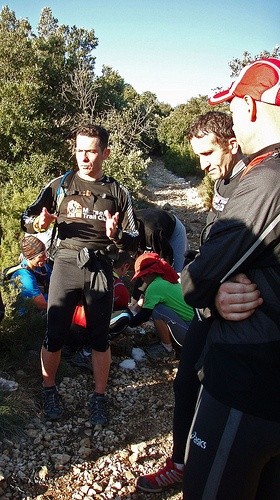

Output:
xmin=208 ymin=57 xmax=280 ymax=107
xmin=21 ymin=235 xmax=46 ymax=260
xmin=131 ymin=252 xmax=180 ymax=284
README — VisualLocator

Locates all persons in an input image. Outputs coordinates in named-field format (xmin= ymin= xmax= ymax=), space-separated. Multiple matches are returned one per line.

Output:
xmin=135 ymin=57 xmax=280 ymax=500
xmin=0 ymin=124 xmax=194 ymax=429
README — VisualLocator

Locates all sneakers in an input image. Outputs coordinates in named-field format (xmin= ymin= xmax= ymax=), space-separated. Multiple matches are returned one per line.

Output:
xmin=90 ymin=392 xmax=110 ymax=427
xmin=144 ymin=342 xmax=176 ymax=362
xmin=136 ymin=457 xmax=186 ymax=493
xmin=42 ymin=385 xmax=65 ymax=420
xmin=0 ymin=376 xmax=18 ymax=393
xmin=70 ymin=348 xmax=92 ymax=371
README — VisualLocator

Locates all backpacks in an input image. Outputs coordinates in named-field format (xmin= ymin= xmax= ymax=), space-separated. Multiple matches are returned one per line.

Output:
xmin=2 ymin=266 xmax=47 ymax=295
